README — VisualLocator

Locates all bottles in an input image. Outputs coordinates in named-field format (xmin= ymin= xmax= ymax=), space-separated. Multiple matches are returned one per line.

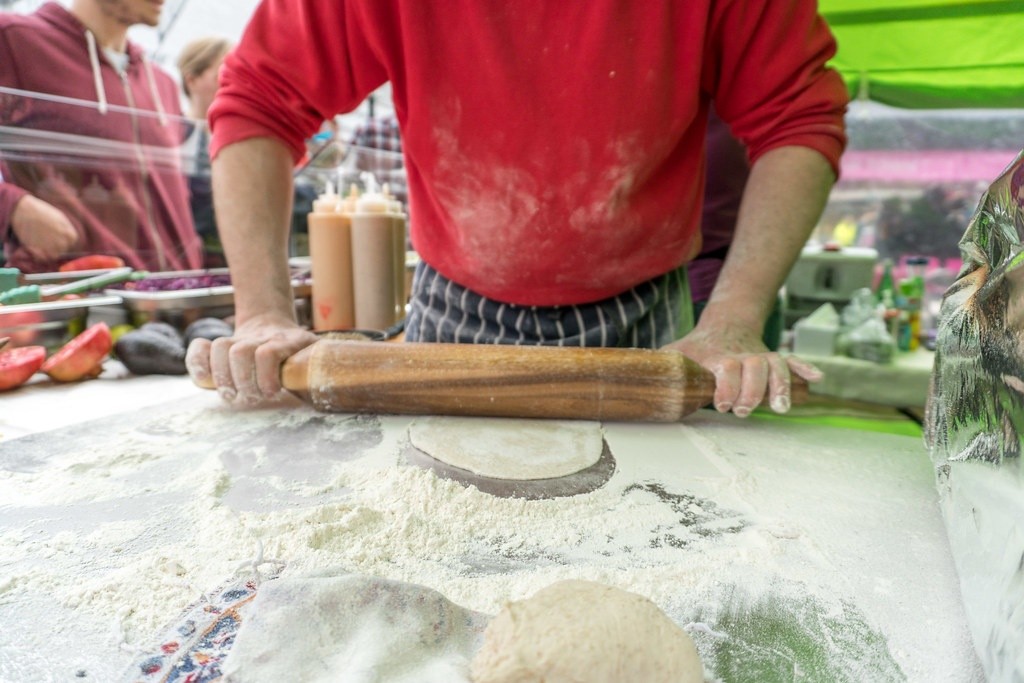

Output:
xmin=875 ymin=259 xmax=898 ymax=307
xmin=308 ymin=175 xmax=407 ymax=332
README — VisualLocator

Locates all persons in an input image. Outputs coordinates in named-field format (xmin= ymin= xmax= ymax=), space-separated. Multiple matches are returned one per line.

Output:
xmin=828 ymin=180 xmax=973 ymax=266
xmin=0 ymin=0 xmax=407 ymax=328
xmin=925 ymin=149 xmax=1024 ymax=683
xmin=186 ymin=0 xmax=849 ymax=429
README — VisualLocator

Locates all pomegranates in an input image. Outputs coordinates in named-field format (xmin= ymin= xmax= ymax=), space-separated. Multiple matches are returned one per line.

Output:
xmin=0 ymin=319 xmax=115 ymax=394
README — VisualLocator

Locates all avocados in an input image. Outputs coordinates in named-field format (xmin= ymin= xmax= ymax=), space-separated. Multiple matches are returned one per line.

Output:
xmin=113 ymin=317 xmax=243 ymax=378
